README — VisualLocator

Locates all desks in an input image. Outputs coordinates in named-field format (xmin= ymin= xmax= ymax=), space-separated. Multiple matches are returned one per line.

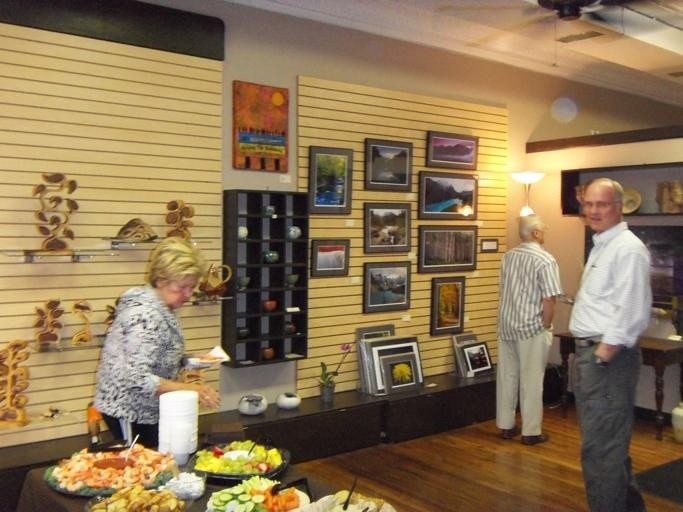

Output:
xmin=553 ymin=331 xmax=683 ymax=441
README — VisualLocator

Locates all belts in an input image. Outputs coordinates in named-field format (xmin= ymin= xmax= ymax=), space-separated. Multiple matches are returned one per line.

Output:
xmin=574 ymin=338 xmax=593 ymax=347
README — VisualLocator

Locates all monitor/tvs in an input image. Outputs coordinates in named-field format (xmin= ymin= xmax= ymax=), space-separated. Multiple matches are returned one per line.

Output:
xmin=581 ymin=223 xmax=683 ymax=315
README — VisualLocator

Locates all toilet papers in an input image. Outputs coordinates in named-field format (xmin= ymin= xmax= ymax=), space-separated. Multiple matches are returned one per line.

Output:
xmin=158 ymin=391 xmax=199 ymax=454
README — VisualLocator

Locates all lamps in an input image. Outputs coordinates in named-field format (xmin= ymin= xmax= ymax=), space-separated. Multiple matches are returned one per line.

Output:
xmin=515 ymin=171 xmax=545 ymax=216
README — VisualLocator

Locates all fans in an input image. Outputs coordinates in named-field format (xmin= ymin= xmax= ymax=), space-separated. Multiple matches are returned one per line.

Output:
xmin=433 ymin=0 xmax=682 ymax=49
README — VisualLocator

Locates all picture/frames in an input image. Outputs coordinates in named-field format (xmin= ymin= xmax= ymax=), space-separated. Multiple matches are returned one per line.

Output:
xmin=355 ymin=131 xmax=497 ymax=397
xmin=309 ymin=146 xmax=353 ymax=215
xmin=311 ymin=239 xmax=350 ymax=277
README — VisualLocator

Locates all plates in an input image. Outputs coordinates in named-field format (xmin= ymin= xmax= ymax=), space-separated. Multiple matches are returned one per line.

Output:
xmin=188 ymin=442 xmax=290 ymax=482
xmin=206 ymin=488 xmax=310 ymax=511
xmin=620 ymin=188 xmax=642 ymax=215
xmin=147 ymin=471 xmax=208 ymax=501
xmin=84 ymin=489 xmax=196 ymax=512
xmin=86 ymin=439 xmax=157 ymax=452
xmin=43 ymin=457 xmax=179 ymax=498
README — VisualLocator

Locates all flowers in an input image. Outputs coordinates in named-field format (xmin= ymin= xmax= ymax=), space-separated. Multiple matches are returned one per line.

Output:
xmin=321 ymin=341 xmax=355 ymax=386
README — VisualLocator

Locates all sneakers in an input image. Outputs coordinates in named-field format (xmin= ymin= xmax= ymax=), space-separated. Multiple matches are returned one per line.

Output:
xmin=522 ymin=433 xmax=548 ymax=445
xmin=503 ymin=427 xmax=520 ymax=439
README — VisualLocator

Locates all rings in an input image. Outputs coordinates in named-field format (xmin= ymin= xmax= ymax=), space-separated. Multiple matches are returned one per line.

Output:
xmin=204 ymin=395 xmax=209 ymax=399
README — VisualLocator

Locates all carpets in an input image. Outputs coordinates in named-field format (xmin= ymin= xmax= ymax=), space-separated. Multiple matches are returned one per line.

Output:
xmin=632 ymin=456 xmax=682 ymax=505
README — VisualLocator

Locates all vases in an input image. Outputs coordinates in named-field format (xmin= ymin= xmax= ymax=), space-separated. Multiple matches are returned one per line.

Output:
xmin=320 ymin=387 xmax=335 ymax=402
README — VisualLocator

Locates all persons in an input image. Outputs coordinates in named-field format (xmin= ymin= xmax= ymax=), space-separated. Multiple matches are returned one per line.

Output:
xmin=93 ymin=237 xmax=219 ymax=448
xmin=495 ymin=214 xmax=565 ymax=446
xmin=570 ymin=177 xmax=652 ymax=512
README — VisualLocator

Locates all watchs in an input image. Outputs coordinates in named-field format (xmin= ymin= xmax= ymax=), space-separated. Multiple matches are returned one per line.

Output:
xmin=591 ymin=354 xmax=607 ymax=367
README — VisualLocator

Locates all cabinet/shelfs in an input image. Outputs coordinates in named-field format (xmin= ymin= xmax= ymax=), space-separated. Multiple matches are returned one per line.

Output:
xmin=222 ymin=189 xmax=309 ymax=369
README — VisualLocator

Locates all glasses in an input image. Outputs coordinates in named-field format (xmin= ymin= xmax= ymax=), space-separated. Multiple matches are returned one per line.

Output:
xmin=584 ymin=199 xmax=619 ymax=209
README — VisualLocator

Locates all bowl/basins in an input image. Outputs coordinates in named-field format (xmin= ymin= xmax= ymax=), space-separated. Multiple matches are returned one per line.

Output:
xmin=158 ymin=389 xmax=198 ymax=465
